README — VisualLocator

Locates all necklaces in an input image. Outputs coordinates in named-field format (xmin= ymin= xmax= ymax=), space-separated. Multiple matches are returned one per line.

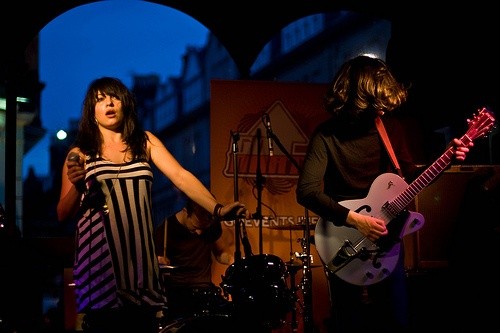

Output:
xmin=94 ymin=144 xmax=129 ymax=215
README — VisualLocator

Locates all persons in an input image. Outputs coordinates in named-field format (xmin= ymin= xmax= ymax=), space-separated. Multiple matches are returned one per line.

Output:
xmin=56 ymin=77 xmax=250 ymax=333
xmin=294 ymin=55 xmax=473 ymax=333
xmin=152 ymin=192 xmax=235 ymax=313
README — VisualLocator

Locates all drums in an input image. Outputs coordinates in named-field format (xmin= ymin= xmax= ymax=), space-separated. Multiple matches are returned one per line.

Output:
xmin=159 ymin=311 xmax=231 ymax=333
xmin=224 ymin=254 xmax=291 ymax=327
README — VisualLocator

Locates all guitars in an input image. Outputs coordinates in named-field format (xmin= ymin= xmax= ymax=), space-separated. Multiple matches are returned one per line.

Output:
xmin=315 ymin=107 xmax=495 ymax=286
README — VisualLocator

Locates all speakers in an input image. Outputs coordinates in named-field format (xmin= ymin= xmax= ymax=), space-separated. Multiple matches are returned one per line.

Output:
xmin=404 ymin=166 xmax=500 ymax=276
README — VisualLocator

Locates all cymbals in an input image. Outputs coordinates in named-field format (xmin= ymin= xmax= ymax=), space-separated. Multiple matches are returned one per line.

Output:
xmin=266 ymin=223 xmax=316 ymax=230
xmin=285 ymin=260 xmax=324 ymax=273
xmin=158 ymin=265 xmax=178 ymax=270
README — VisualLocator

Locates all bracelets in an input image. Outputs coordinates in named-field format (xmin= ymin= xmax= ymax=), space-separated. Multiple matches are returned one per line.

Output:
xmin=212 ymin=202 xmax=225 ymax=222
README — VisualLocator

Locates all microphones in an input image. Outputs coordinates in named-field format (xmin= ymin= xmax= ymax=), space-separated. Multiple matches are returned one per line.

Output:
xmin=266 ymin=113 xmax=273 ymax=156
xmin=242 ymin=221 xmax=252 ymax=254
xmin=68 ymin=152 xmax=87 ymax=194
